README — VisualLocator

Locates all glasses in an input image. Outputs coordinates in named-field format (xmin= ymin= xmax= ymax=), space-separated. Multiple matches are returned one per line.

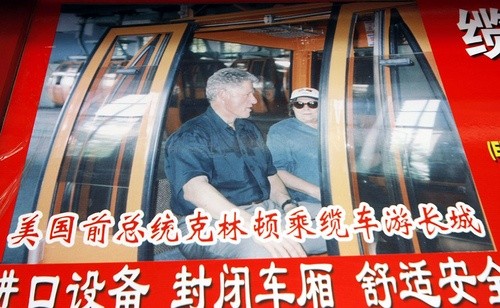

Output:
xmin=291 ymin=101 xmax=319 ymax=109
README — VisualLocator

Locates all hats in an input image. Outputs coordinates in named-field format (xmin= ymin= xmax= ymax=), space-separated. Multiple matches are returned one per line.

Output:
xmin=290 ymin=87 xmax=319 ymax=100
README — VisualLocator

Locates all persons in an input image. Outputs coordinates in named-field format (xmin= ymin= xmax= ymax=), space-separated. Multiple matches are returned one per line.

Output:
xmin=265 ymin=87 xmax=320 ymax=201
xmin=163 ymin=66 xmax=380 ymax=262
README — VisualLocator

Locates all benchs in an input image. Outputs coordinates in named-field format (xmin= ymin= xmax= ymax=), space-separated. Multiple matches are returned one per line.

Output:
xmin=157 ymin=179 xmax=327 ymax=259
